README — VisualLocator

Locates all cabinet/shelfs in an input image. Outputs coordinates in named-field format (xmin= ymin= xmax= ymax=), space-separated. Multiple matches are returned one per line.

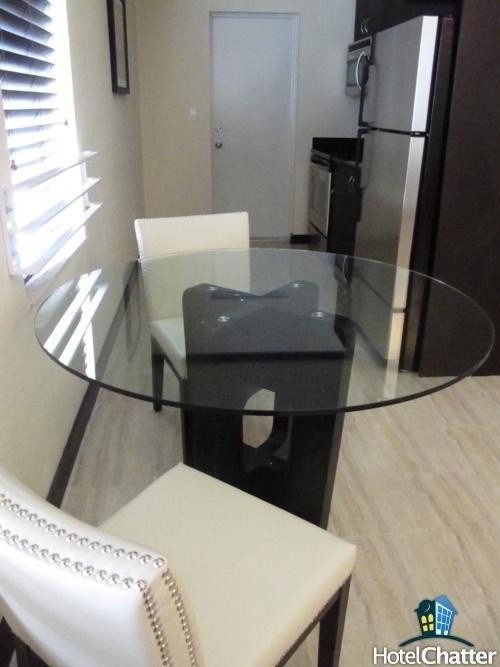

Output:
xmin=355 ymin=0 xmax=385 ymax=40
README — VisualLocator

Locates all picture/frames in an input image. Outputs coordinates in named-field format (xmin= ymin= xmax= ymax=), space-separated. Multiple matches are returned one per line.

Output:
xmin=107 ymin=0 xmax=130 ymax=95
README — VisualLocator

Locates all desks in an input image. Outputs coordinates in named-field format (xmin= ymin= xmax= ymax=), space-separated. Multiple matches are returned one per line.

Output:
xmin=34 ymin=247 xmax=495 ymax=529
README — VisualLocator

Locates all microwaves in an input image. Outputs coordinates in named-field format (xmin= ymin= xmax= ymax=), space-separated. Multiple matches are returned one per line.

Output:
xmin=346 ymin=36 xmax=373 ymax=98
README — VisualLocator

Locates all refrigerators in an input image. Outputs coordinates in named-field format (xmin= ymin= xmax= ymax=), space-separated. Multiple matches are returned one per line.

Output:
xmin=346 ymin=14 xmax=453 ymax=367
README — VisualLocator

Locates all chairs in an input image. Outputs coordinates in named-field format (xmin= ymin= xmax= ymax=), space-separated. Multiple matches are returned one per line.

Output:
xmin=0 ymin=461 xmax=360 ymax=665
xmin=134 ymin=212 xmax=252 ymax=410
